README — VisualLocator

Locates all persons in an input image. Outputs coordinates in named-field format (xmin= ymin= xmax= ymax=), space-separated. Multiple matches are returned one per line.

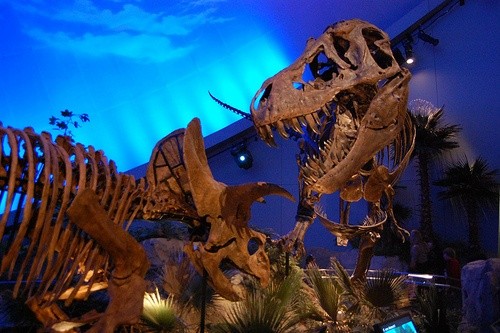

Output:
xmin=394 ymin=224 xmax=461 ymax=278
xmin=305 ymin=255 xmax=322 ymax=285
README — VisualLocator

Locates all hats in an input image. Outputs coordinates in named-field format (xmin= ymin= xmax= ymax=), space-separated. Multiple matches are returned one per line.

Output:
xmin=442 ymin=250 xmax=455 ymax=259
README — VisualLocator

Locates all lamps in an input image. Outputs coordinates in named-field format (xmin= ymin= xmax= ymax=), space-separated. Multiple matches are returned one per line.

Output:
xmin=405 ymin=44 xmax=413 ymax=65
xmin=229 ymin=141 xmax=254 ymax=170
xmin=417 ymin=30 xmax=440 ymax=46
